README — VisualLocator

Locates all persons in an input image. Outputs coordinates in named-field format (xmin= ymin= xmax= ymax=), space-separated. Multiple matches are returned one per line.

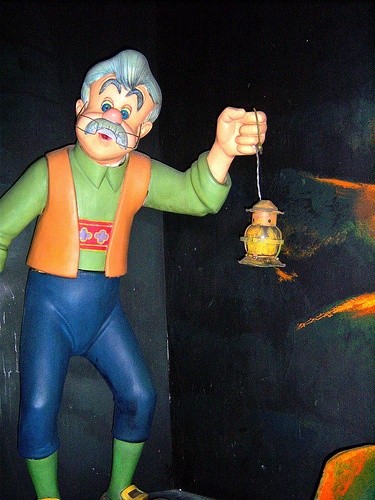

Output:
xmin=0 ymin=49 xmax=269 ymax=500
